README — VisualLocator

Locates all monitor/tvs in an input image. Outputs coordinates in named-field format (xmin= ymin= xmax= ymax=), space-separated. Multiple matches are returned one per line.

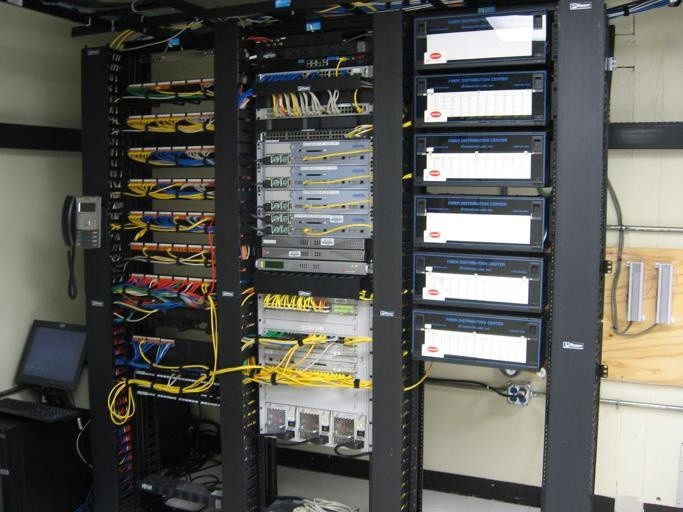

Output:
xmin=14 ymin=319 xmax=88 ymax=408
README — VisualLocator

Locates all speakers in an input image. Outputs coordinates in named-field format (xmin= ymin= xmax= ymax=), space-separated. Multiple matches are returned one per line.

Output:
xmin=626 ymin=261 xmax=644 ymax=321
xmin=655 ymin=263 xmax=673 ymax=324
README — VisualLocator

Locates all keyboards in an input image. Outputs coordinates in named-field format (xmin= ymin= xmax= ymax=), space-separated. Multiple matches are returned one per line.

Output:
xmin=0 ymin=398 xmax=80 ymax=425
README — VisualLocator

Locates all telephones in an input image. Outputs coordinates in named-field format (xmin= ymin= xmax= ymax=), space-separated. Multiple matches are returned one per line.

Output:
xmin=61 ymin=194 xmax=101 ymax=249
